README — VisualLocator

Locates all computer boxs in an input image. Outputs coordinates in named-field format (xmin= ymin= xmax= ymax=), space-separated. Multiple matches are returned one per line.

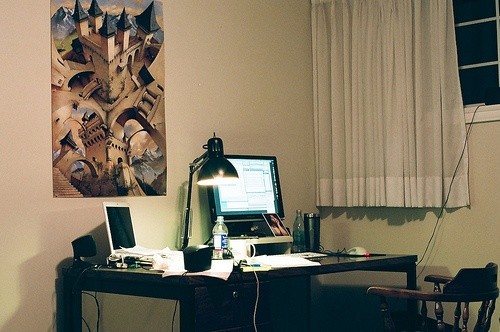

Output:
xmin=227 ymin=235 xmax=295 ymax=258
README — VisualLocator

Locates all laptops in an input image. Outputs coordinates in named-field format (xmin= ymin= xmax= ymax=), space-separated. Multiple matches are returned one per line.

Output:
xmin=102 ymin=201 xmax=164 ymax=263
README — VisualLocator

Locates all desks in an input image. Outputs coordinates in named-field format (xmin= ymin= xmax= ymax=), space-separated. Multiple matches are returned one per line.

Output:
xmin=56 ymin=251 xmax=418 ymax=331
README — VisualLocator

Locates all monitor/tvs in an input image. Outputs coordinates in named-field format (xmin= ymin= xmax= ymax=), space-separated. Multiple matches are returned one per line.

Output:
xmin=207 ymin=155 xmax=285 ymax=236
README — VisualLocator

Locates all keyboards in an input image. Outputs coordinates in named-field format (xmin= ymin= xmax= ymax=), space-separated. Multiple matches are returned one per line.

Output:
xmin=285 ymin=252 xmax=328 ymax=258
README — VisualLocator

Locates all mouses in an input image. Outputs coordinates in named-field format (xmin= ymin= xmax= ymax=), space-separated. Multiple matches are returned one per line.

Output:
xmin=347 ymin=246 xmax=367 ymax=257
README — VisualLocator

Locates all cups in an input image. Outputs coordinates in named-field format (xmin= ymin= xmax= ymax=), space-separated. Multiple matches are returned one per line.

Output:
xmin=229 ymin=239 xmax=256 ymax=259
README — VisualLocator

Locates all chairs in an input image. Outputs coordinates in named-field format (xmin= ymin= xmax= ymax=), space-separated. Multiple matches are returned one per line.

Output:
xmin=366 ymin=262 xmax=499 ymax=332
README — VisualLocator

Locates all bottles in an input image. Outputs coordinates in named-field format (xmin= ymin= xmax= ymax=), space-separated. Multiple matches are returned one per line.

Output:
xmin=212 ymin=216 xmax=229 ymax=259
xmin=304 ymin=213 xmax=321 ymax=252
xmin=293 ymin=209 xmax=306 ymax=252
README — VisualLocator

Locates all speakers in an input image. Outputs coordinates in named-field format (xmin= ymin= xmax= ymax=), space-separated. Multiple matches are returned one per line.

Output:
xmin=71 ymin=234 xmax=98 ymax=258
xmin=183 ymin=244 xmax=212 ymax=272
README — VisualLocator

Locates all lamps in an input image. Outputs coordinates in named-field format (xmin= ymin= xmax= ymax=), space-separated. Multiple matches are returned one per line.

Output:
xmin=181 ymin=133 xmax=239 ymax=249
